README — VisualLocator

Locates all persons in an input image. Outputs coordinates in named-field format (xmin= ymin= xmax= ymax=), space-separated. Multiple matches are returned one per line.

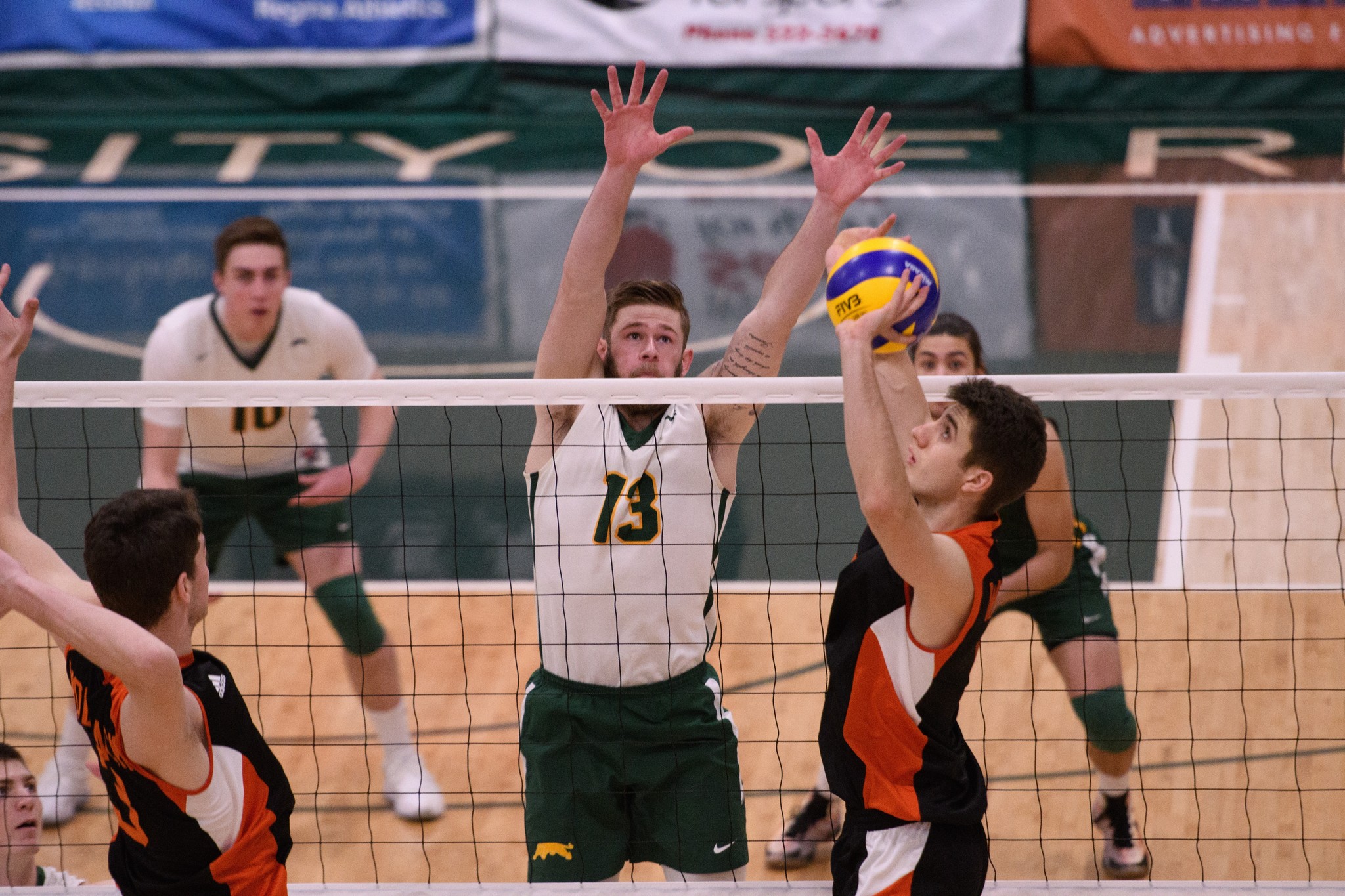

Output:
xmin=518 ymin=61 xmax=908 ymax=882
xmin=0 ymin=263 xmax=296 ymax=896
xmin=766 ymin=314 xmax=1152 ymax=882
xmin=818 ymin=213 xmax=1046 ymax=896
xmin=0 ymin=741 xmax=86 ymax=887
xmin=37 ymin=213 xmax=446 ymax=822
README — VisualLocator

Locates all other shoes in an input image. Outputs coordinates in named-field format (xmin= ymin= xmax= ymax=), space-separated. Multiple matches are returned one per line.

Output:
xmin=34 ymin=754 xmax=88 ymax=828
xmin=382 ymin=749 xmax=445 ymax=820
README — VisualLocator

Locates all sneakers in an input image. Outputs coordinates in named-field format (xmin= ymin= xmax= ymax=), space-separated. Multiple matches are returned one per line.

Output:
xmin=1091 ymin=789 xmax=1149 ymax=879
xmin=764 ymin=789 xmax=841 ymax=870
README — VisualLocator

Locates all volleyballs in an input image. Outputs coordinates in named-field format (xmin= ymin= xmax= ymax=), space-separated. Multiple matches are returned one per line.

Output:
xmin=825 ymin=236 xmax=941 ymax=354
